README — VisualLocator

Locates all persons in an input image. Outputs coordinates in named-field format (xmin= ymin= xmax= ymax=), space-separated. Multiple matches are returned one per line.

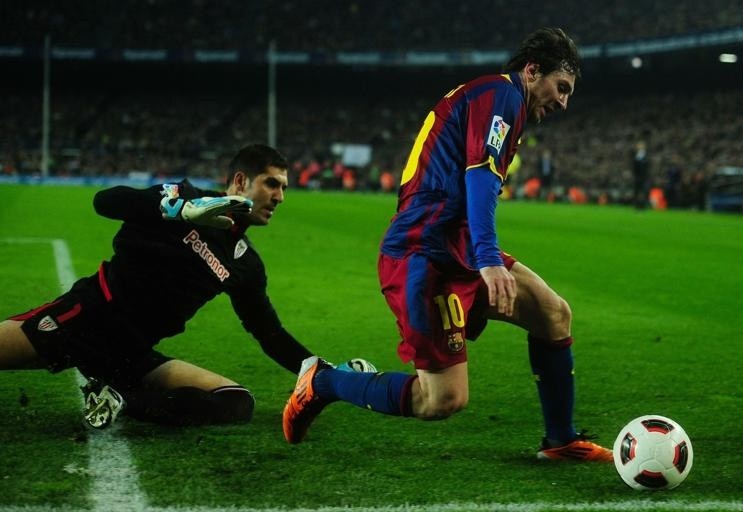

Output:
xmin=0 ymin=145 xmax=379 ymax=426
xmin=281 ymin=26 xmax=613 ymax=462
xmin=1 ymin=2 xmax=742 ymax=210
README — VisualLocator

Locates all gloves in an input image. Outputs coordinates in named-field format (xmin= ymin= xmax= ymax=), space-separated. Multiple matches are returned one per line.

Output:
xmin=336 ymin=358 xmax=377 ymax=372
xmin=159 ymin=196 xmax=253 ymax=229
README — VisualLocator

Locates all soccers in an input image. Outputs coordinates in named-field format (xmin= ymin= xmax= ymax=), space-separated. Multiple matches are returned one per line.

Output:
xmin=613 ymin=415 xmax=693 ymax=491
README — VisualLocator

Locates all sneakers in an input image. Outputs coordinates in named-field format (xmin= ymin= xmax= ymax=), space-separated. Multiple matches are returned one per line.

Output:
xmin=535 ymin=430 xmax=614 ymax=463
xmin=282 ymin=356 xmax=332 ymax=444
xmin=80 ymin=377 xmax=128 ymax=430
